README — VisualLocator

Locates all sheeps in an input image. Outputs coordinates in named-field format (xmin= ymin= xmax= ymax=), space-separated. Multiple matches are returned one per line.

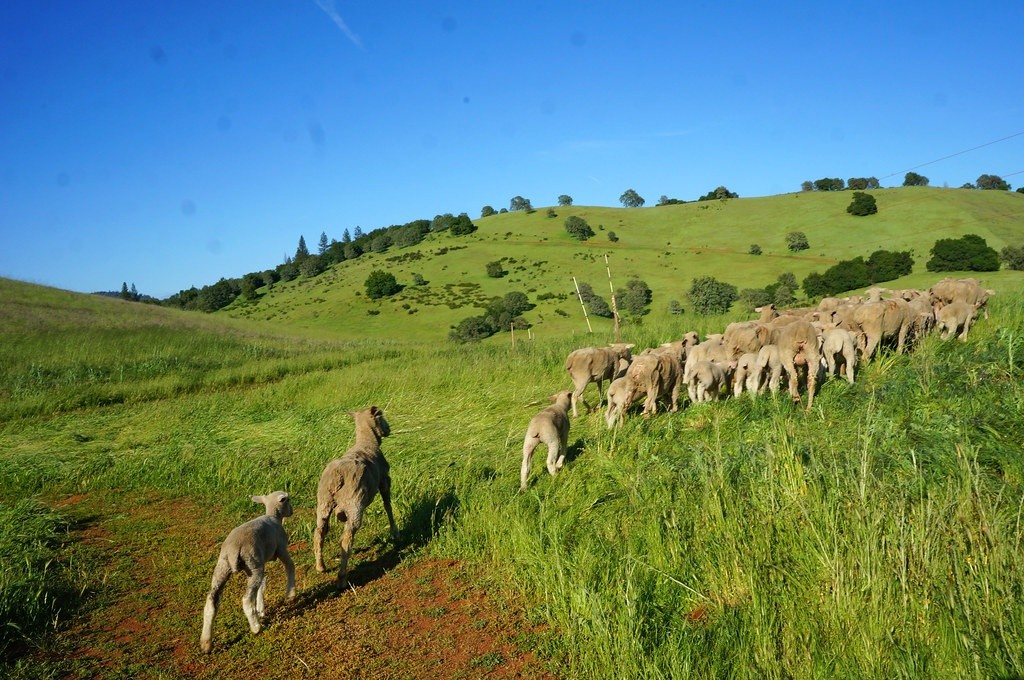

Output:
xmin=199 ymin=491 xmax=296 ymax=653
xmin=314 ymin=406 xmax=401 ymax=589
xmin=520 ymin=276 xmax=995 ymax=495
